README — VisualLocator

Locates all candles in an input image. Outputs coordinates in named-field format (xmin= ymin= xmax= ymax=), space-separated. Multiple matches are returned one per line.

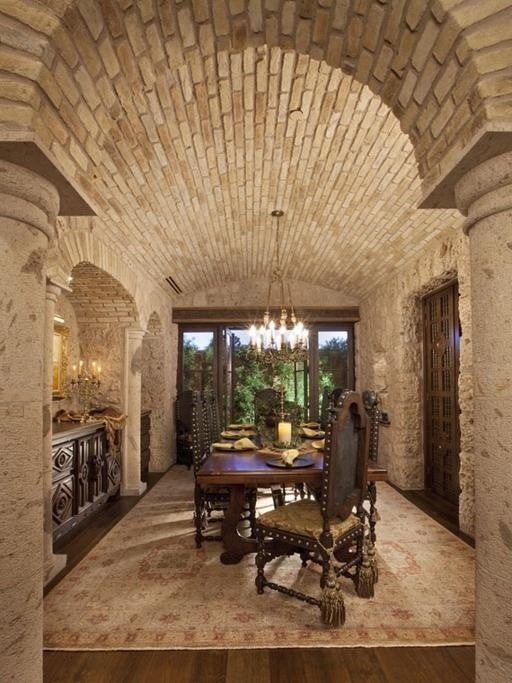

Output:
xmin=278 ymin=421 xmax=292 ymax=444
xmin=72 ymin=358 xmax=100 ymax=381
xmin=248 ymin=318 xmax=309 ymax=349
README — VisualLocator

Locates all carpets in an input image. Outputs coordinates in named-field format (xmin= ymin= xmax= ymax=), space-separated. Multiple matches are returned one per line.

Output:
xmin=42 ymin=461 xmax=477 ymax=652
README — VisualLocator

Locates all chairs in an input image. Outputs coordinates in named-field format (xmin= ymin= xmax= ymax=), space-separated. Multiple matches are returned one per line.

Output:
xmin=253 ymin=389 xmax=284 ymax=428
xmin=319 ymin=384 xmax=378 ymax=546
xmin=251 ymin=391 xmax=376 ymax=627
xmin=189 ymin=387 xmax=257 ymax=548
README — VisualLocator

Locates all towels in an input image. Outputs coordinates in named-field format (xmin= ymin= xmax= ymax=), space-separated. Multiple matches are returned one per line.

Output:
xmin=228 ymin=420 xmax=325 ymax=466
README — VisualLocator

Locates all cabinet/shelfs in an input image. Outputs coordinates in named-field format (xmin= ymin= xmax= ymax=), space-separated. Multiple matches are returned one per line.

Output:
xmin=52 ymin=415 xmax=121 ymax=545
xmin=141 ymin=409 xmax=152 ymax=481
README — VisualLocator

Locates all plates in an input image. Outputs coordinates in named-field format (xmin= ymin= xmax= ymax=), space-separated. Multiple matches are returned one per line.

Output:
xmin=212 ymin=422 xmax=254 ymax=452
xmin=266 ymin=456 xmax=315 ymax=468
xmin=299 ymin=425 xmax=324 ymax=451
